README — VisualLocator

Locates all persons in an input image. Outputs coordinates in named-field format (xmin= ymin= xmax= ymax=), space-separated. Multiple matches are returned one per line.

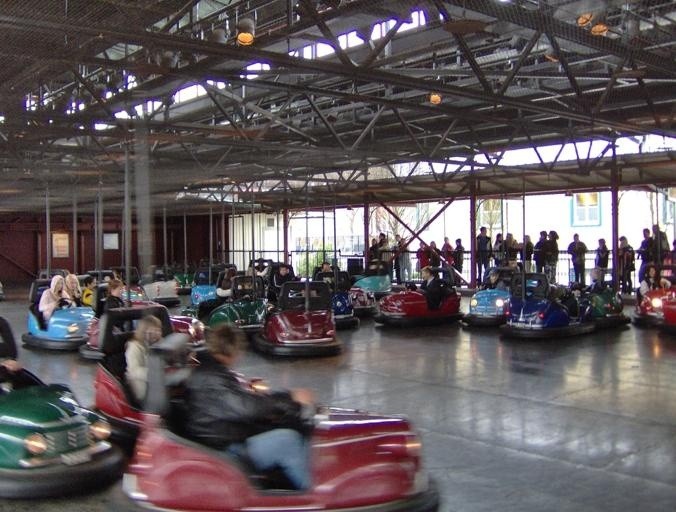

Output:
xmin=476 ymin=226 xmax=492 ymax=286
xmin=416 ymin=237 xmax=464 ymax=288
xmin=617 ymin=225 xmax=675 ymax=297
xmin=177 ymin=324 xmax=316 ymax=490
xmin=594 ymin=239 xmax=609 ymax=280
xmin=568 ymin=233 xmax=586 ymax=284
xmin=369 ymin=233 xmax=413 ymax=280
xmin=493 ymin=230 xmax=559 ymax=284
xmin=273 ymin=264 xmax=296 ymax=297
xmin=38 ymin=273 xmax=125 ymax=328
xmin=217 ymin=268 xmax=240 ymax=302
xmin=417 ymin=266 xmax=606 ymax=317
xmin=255 ymin=258 xmax=267 ymax=273
xmin=124 ymin=315 xmax=163 ymax=405
xmin=315 ymin=262 xmax=332 ymax=280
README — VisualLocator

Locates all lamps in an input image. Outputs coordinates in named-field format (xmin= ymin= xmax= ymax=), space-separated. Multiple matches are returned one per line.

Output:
xmin=575 ymin=12 xmax=593 ymax=26
xmin=591 ymin=14 xmax=608 ymax=35
xmin=237 ymin=18 xmax=256 ymax=47
xmin=430 ymin=79 xmax=445 ymax=104
xmin=205 ymin=28 xmax=230 ymax=44
